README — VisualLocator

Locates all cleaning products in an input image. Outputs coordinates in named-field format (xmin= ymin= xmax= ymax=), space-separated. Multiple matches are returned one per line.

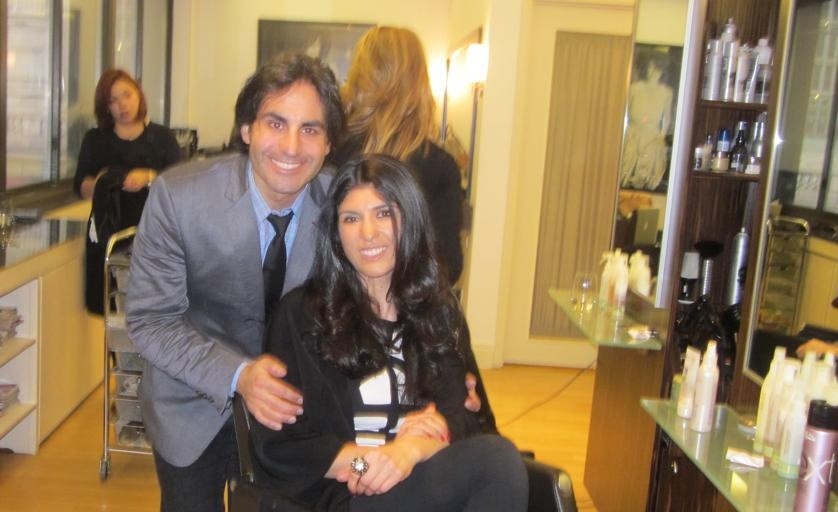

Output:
xmin=722 ymin=217 xmax=749 ymax=306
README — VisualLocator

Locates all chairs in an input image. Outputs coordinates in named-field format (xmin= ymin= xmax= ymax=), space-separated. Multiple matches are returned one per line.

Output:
xmin=225 ymin=389 xmax=582 ymax=512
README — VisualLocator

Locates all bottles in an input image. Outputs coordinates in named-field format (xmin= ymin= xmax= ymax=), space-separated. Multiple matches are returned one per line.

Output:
xmin=753 ymin=340 xmax=838 ymax=512
xmin=726 ymin=225 xmax=751 ymax=309
xmin=701 ymin=22 xmax=773 ymax=109
xmin=690 ymin=339 xmax=720 ymax=432
xmin=613 ymin=258 xmax=628 ymax=316
xmin=635 ymin=257 xmax=650 ymax=298
xmin=690 ymin=122 xmax=765 ymax=174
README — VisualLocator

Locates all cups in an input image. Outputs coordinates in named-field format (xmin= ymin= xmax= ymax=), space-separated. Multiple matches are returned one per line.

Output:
xmin=1 ymin=213 xmax=16 ymax=250
xmin=572 ymin=270 xmax=597 ymax=312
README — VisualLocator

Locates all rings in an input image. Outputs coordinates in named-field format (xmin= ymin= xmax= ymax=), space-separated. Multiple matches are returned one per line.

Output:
xmin=351 ymin=456 xmax=369 ymax=474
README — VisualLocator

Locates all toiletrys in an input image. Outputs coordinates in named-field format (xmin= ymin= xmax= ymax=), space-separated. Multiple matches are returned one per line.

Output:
xmin=692 ymin=109 xmax=766 ymax=173
xmin=698 ymin=18 xmax=774 ymax=103
xmin=674 ymin=341 xmax=838 ymax=477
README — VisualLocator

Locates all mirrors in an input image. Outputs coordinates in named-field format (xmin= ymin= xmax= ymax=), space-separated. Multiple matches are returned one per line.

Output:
xmin=605 ymin=0 xmax=698 ymax=315
xmin=737 ymin=1 xmax=838 ymax=406
xmin=439 ymin=23 xmax=484 ymax=209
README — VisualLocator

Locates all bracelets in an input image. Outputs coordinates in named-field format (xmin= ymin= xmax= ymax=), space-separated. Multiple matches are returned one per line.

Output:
xmin=147 ymin=167 xmax=152 ymax=187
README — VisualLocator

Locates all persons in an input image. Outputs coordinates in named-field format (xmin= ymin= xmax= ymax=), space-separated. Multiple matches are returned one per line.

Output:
xmin=338 ymin=26 xmax=463 ymax=288
xmin=124 ymin=50 xmax=481 ymax=512
xmin=249 ymin=153 xmax=531 ymax=512
xmin=620 ymin=51 xmax=675 ymax=191
xmin=73 ymin=69 xmax=180 ymax=316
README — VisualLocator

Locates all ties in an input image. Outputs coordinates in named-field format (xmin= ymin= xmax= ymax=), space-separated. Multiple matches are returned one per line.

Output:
xmin=263 ymin=212 xmax=293 ymax=324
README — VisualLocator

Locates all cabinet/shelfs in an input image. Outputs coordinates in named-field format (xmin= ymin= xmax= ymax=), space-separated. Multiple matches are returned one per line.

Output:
xmin=637 ymin=0 xmax=797 ymax=512
xmin=1 ymin=211 xmax=114 ymax=457
